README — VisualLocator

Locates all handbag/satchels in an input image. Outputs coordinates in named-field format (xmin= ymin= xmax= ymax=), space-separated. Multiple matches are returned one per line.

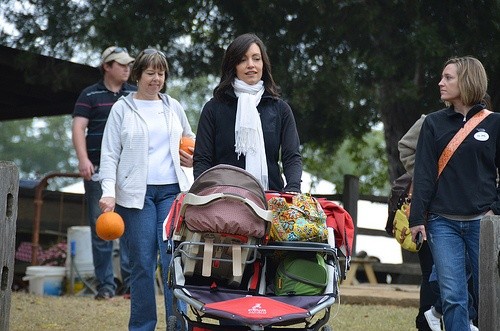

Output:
xmin=392 ymin=203 xmax=422 ymax=252
xmin=274 ymin=260 xmax=327 ymax=296
xmin=267 ymin=193 xmax=328 ymax=242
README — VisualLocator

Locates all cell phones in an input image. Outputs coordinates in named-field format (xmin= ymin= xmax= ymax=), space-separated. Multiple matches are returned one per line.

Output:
xmin=415 ymin=231 xmax=422 ymax=251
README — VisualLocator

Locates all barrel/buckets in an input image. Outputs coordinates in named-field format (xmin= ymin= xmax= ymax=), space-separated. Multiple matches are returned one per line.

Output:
xmin=22 ymin=265 xmax=66 ymax=296
xmin=65 ymin=225 xmax=95 ymax=279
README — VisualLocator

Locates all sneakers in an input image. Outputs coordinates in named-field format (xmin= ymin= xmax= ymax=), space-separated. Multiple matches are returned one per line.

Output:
xmin=470 ymin=321 xmax=480 ymax=331
xmin=425 ymin=306 xmax=442 ymax=331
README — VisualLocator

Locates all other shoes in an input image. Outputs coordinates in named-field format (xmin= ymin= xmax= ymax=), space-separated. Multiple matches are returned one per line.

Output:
xmin=95 ymin=290 xmax=113 ymax=299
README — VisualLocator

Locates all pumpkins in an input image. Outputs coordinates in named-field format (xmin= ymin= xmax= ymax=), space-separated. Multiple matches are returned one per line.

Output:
xmin=179 ymin=137 xmax=194 ymax=155
xmin=96 ymin=212 xmax=125 ymax=240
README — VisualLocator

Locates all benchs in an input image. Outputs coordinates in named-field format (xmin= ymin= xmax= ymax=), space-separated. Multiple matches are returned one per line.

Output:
xmin=340 ymin=256 xmax=379 ymax=285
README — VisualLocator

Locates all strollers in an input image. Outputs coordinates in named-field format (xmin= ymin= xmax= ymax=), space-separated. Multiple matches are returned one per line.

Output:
xmin=163 ymin=164 xmax=354 ymax=331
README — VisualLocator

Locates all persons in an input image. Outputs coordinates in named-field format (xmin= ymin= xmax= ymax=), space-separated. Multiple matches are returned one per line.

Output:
xmin=397 ymin=114 xmax=444 ymax=330
xmin=194 ymin=33 xmax=302 ymax=195
xmin=100 ymin=49 xmax=196 ymax=331
xmin=73 ymin=46 xmax=131 ymax=298
xmin=408 ymin=56 xmax=500 ymax=331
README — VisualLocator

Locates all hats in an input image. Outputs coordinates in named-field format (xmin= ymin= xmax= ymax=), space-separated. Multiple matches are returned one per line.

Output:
xmin=102 ymin=46 xmax=135 ymax=65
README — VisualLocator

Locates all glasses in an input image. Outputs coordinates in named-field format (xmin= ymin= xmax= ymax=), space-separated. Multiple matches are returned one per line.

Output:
xmin=139 ymin=49 xmax=166 ymax=60
xmin=103 ymin=48 xmax=127 ymax=61
